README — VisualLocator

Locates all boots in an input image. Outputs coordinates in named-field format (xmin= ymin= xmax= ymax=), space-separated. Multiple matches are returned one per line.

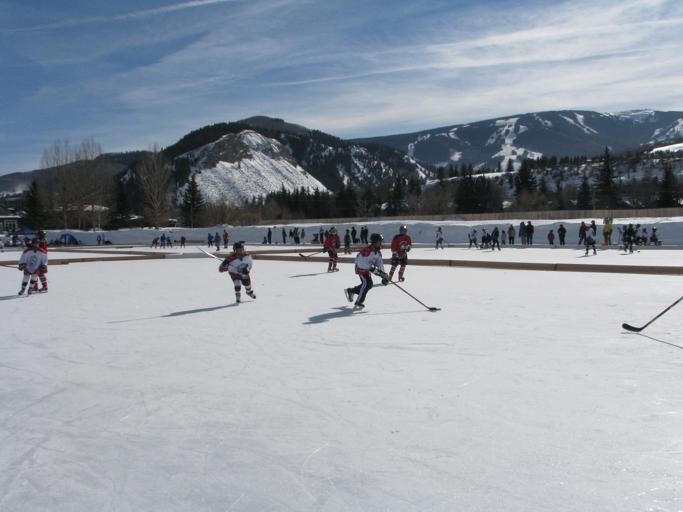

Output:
xmin=246 ymin=291 xmax=256 ymax=299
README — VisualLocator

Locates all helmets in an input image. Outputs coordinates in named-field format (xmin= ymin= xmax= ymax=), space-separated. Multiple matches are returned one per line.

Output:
xmin=370 ymin=234 xmax=383 ymax=243
xmin=400 ymin=225 xmax=407 ymax=231
xmin=32 ymin=230 xmax=47 ymax=244
xmin=233 ymin=243 xmax=244 ymax=250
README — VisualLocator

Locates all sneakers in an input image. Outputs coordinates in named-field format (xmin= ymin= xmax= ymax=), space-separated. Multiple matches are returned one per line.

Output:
xmin=19 ymin=287 xmax=48 ymax=295
xmin=328 ymin=268 xmax=404 ymax=308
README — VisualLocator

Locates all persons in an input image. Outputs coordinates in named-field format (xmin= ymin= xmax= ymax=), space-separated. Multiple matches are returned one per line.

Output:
xmin=219 ymin=241 xmax=256 ymax=304
xmin=208 ymin=229 xmax=228 ymax=251
xmin=281 ymin=227 xmax=330 ymax=244
xmin=389 ymin=225 xmax=412 ymax=281
xmin=151 ymin=233 xmax=172 ymax=248
xmin=519 ymin=221 xmax=534 ymax=245
xmin=344 ymin=226 xmax=368 ymax=254
xmin=324 ymin=226 xmax=340 ymax=273
xmin=345 ymin=232 xmax=389 ymax=308
xmin=435 ymin=227 xmax=443 ymax=249
xmin=181 ymin=236 xmax=186 ymax=246
xmin=267 ymin=228 xmax=272 ymax=243
xmin=468 ymin=225 xmax=515 ymax=250
xmin=18 ymin=230 xmax=48 ymax=295
xmin=578 ymin=220 xmax=659 ymax=256
xmin=547 ymin=224 xmax=566 ymax=245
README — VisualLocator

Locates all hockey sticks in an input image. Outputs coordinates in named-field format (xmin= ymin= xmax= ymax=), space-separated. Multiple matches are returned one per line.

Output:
xmin=622 ymin=296 xmax=683 ymax=332
xmin=612 ymin=231 xmax=621 ymax=245
xmin=382 ymin=275 xmax=441 ymax=310
xmin=198 ymin=246 xmax=239 ymax=269
xmin=299 ymin=250 xmax=324 ymax=258
